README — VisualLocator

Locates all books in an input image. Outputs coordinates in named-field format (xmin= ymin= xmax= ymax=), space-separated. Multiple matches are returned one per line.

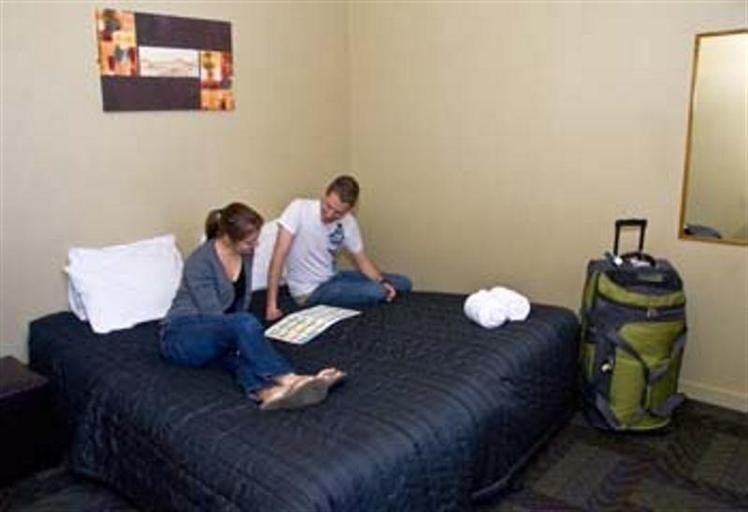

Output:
xmin=264 ymin=304 xmax=363 ymax=345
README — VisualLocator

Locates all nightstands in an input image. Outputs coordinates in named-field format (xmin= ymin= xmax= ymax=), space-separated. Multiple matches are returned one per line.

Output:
xmin=0 ymin=354 xmax=52 ymax=493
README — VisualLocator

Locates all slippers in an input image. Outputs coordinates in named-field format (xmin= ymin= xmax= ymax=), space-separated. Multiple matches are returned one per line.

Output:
xmin=259 ymin=376 xmax=327 ymax=410
xmin=286 ymin=367 xmax=347 ymax=393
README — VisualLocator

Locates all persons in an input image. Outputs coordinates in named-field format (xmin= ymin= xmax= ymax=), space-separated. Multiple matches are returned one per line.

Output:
xmin=265 ymin=175 xmax=414 ymax=322
xmin=159 ymin=201 xmax=347 ymax=411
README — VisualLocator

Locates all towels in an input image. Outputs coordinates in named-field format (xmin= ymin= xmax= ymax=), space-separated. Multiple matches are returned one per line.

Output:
xmin=463 ymin=286 xmax=532 ymax=330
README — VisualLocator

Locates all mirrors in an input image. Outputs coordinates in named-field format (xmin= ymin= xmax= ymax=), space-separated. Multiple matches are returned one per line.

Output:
xmin=677 ymin=28 xmax=748 ymax=247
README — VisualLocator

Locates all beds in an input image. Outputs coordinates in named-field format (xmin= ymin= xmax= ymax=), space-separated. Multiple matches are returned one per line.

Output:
xmin=27 ymin=272 xmax=581 ymax=511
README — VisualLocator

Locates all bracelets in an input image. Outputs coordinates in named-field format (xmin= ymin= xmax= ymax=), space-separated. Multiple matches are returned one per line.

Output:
xmin=377 ymin=277 xmax=394 ymax=285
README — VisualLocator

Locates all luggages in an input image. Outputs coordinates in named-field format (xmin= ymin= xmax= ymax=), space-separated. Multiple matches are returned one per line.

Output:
xmin=579 ymin=218 xmax=689 ymax=430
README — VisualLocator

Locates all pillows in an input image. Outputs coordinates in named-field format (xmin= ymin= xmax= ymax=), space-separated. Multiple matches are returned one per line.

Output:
xmin=61 ymin=232 xmax=185 ymax=337
xmin=197 ymin=217 xmax=287 ymax=293
